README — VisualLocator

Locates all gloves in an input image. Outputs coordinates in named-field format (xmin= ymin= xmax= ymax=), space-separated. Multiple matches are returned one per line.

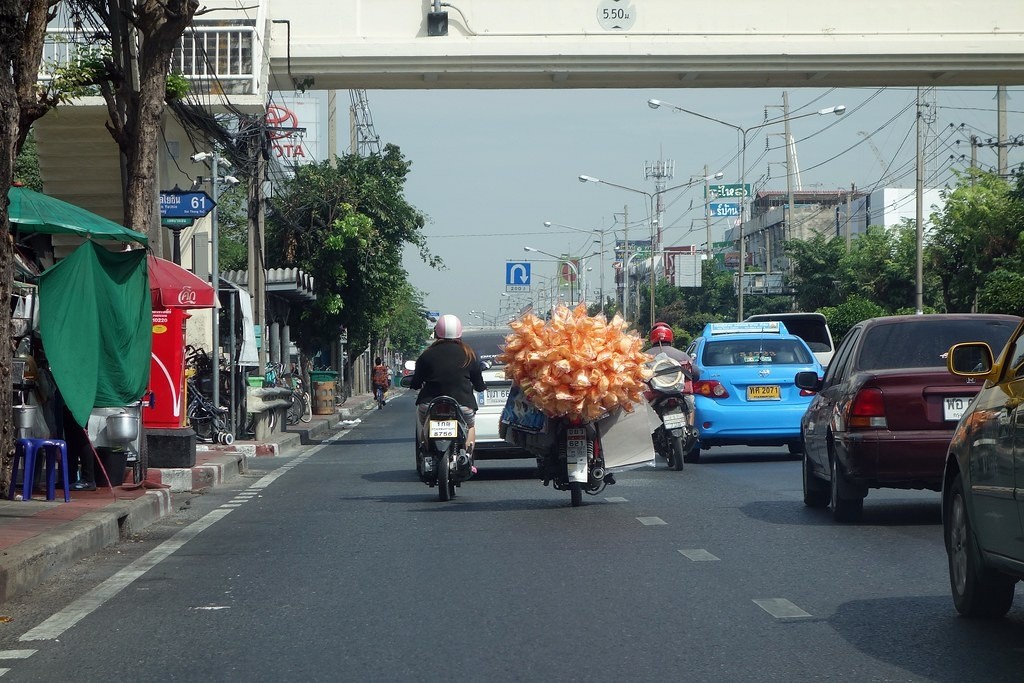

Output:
xmin=484 ymin=381 xmax=487 ymax=389
xmin=411 ymin=384 xmax=422 ymax=390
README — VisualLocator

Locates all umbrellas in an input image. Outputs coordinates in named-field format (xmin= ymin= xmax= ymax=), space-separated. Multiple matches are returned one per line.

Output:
xmin=147 ymin=255 xmax=222 ymax=309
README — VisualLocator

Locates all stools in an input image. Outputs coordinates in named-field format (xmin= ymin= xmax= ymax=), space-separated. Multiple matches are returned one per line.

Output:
xmin=7 ymin=438 xmax=70 ymax=503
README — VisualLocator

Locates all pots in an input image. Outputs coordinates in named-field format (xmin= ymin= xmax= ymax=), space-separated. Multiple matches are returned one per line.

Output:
xmin=107 ymin=410 xmax=137 ymax=440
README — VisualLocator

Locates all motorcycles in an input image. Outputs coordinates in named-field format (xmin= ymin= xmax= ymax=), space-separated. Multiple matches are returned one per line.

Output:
xmin=534 ymin=404 xmax=609 ymax=502
xmin=401 ymin=373 xmax=476 ymax=504
xmin=640 ymin=356 xmax=695 ymax=473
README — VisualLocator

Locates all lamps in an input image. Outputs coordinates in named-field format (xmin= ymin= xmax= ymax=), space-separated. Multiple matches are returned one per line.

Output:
xmin=427 ymin=11 xmax=449 ymax=36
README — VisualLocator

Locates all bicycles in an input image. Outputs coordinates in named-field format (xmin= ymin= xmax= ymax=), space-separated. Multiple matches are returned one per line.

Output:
xmin=375 ymin=387 xmax=384 ymax=409
xmin=185 ymin=344 xmax=351 ymax=447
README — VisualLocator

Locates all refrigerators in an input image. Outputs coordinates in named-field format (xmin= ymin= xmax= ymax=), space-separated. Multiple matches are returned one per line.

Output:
xmin=142 ymin=309 xmax=192 ymax=427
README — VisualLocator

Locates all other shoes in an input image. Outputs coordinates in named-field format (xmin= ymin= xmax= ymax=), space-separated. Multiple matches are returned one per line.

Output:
xmin=381 ymin=400 xmax=386 ymax=405
xmin=471 ymin=466 xmax=477 ymax=473
xmin=374 ymin=396 xmax=378 ymax=400
xmin=688 ymin=425 xmax=699 ymax=434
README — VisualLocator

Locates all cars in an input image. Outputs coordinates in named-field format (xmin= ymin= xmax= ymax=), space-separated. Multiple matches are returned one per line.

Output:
xmin=747 ymin=313 xmax=838 ymax=375
xmin=941 ymin=311 xmax=1023 ymax=608
xmin=686 ymin=321 xmax=823 ymax=463
xmin=793 ymin=312 xmax=1024 ymax=522
xmin=450 ymin=327 xmax=548 ymax=459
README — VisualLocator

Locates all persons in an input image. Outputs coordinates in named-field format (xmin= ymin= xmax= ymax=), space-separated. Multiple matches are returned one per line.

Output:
xmin=370 ymin=357 xmax=393 ymax=405
xmin=410 ymin=314 xmax=487 ymax=474
xmin=53 ymin=387 xmax=97 ymax=492
xmin=640 ymin=322 xmax=700 ymax=434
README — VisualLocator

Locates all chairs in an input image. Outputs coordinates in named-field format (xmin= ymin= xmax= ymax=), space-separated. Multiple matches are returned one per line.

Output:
xmin=707 ymin=353 xmax=735 ymax=364
xmin=774 ymin=352 xmax=794 ymax=363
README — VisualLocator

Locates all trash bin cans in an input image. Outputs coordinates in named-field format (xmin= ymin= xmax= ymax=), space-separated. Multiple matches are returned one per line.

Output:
xmin=395 ymin=376 xmax=401 ymax=387
xmin=308 ymin=372 xmax=339 ymax=409
xmin=313 ymin=381 xmax=335 ymax=415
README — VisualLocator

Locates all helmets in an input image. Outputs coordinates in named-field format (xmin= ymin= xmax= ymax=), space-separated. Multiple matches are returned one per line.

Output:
xmin=649 ymin=322 xmax=673 ymax=348
xmin=435 ymin=314 xmax=461 ymax=338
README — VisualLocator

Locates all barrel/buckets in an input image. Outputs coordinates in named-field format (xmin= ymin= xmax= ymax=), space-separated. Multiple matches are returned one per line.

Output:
xmin=94 ymin=448 xmax=128 ymax=486
xmin=13 ymin=391 xmax=37 ymax=428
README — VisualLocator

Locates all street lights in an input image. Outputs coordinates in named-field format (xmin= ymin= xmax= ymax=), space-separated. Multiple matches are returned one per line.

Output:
xmin=463 ymin=267 xmax=596 ymax=328
xmin=524 ymin=244 xmax=609 ymax=314
xmin=646 ymin=96 xmax=846 ymax=320
xmin=577 ymin=172 xmax=726 ymax=328
xmin=543 ymin=219 xmax=665 ymax=317
